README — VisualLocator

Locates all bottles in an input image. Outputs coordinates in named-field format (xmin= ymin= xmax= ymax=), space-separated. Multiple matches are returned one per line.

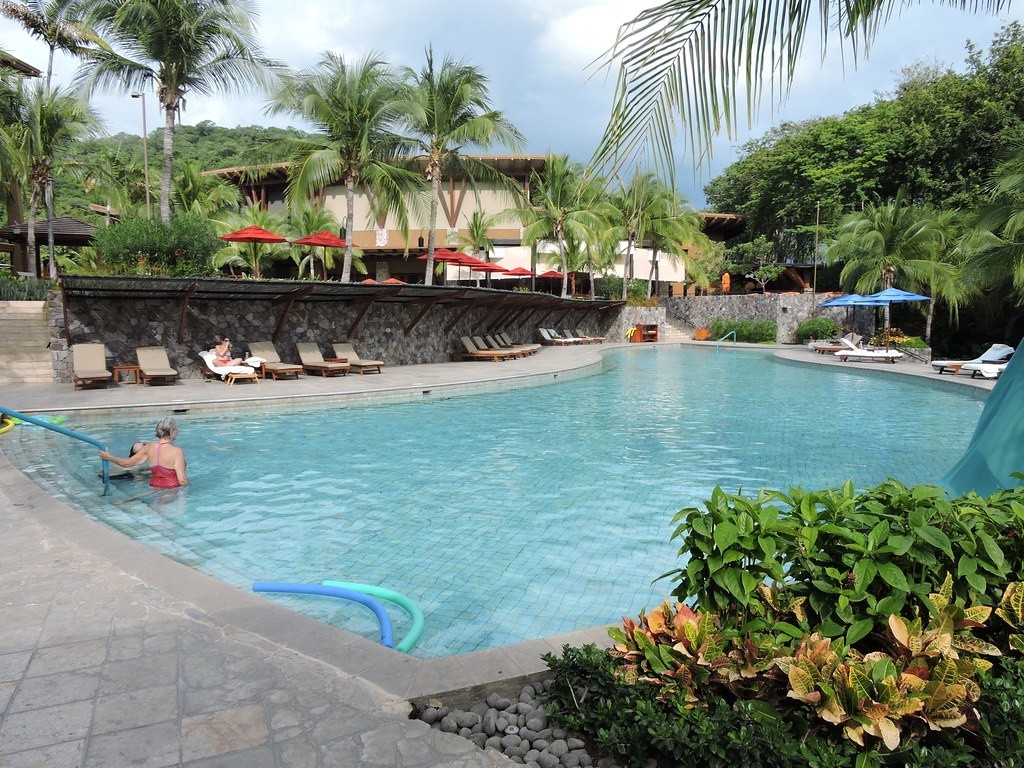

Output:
xmin=228 ymin=343 xmax=233 ymax=347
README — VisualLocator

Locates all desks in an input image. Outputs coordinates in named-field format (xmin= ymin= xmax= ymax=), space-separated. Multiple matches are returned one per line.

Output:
xmin=241 ymin=357 xmax=266 ymax=380
xmin=113 ymin=366 xmax=141 ymax=385
xmin=324 ymin=357 xmax=350 ymax=374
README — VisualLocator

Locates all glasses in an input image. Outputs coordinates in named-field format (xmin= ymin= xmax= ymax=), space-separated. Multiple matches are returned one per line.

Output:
xmin=224 ymin=340 xmax=230 ymax=342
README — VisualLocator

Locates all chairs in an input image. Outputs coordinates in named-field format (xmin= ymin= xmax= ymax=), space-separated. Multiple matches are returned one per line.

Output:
xmin=136 ymin=346 xmax=179 ymax=386
xmin=248 ymin=340 xmax=304 ymax=381
xmin=71 ymin=343 xmax=113 ymax=391
xmin=295 ymin=342 xmax=351 ymax=378
xmin=808 ymin=333 xmax=1015 ymax=380
xmin=450 ymin=328 xmax=606 ymax=362
xmin=198 ymin=348 xmax=260 ymax=387
xmin=332 ymin=343 xmax=384 ymax=376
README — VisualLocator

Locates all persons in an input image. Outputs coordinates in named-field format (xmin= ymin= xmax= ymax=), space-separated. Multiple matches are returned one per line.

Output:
xmin=98 ymin=417 xmax=189 ymax=487
xmin=212 ymin=336 xmax=242 ymax=367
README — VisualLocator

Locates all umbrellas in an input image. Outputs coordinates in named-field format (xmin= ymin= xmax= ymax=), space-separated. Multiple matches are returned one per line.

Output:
xmin=290 ymin=230 xmax=360 ymax=269
xmin=817 ymin=293 xmax=888 ymax=344
xmin=417 ymin=248 xmax=490 ymax=287
xmin=537 ymin=270 xmax=564 ymax=294
xmin=220 ymin=225 xmax=289 ymax=270
xmin=471 ymin=262 xmax=510 ymax=288
xmin=502 ymin=267 xmax=534 ymax=287
xmin=854 ymin=287 xmax=931 ymax=353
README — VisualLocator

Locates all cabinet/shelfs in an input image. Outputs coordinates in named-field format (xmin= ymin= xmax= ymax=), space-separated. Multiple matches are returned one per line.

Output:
xmin=630 ymin=323 xmax=658 ymax=343
